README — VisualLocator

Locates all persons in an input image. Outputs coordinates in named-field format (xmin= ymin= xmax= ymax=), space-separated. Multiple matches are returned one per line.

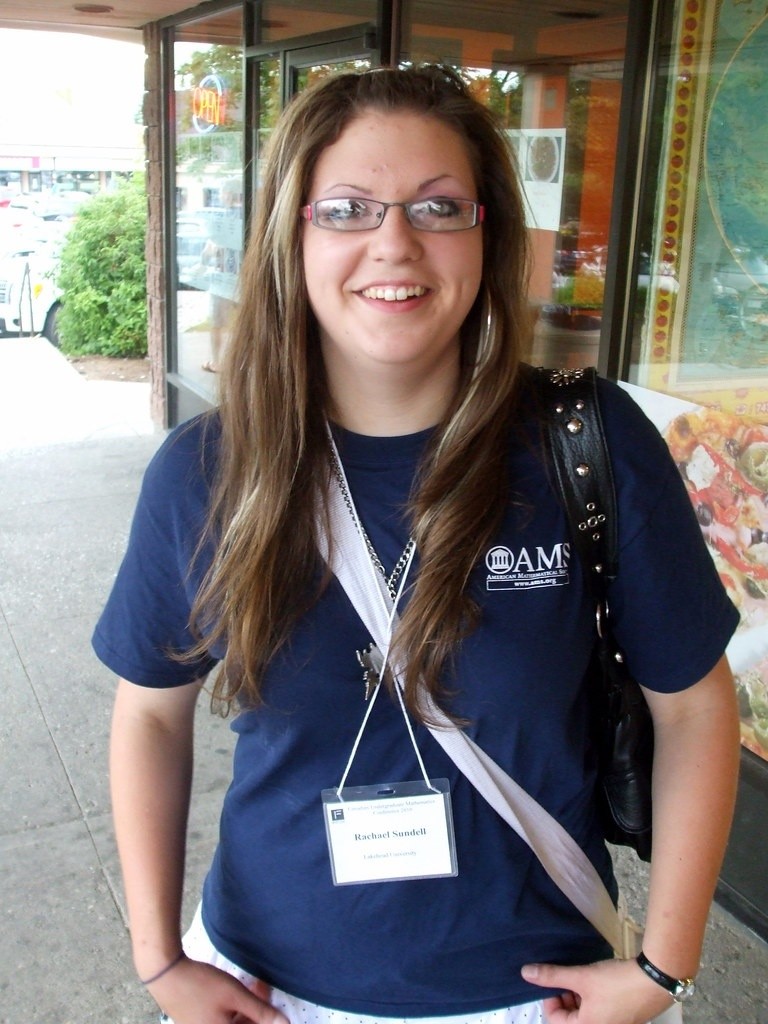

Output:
xmin=92 ymin=65 xmax=743 ymax=1023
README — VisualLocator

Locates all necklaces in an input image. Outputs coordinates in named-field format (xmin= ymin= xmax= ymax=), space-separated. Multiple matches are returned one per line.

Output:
xmin=322 ymin=388 xmax=423 ymax=704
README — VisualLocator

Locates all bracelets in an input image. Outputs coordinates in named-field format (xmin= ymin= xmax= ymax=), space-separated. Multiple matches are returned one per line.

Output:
xmin=141 ymin=950 xmax=186 ymax=985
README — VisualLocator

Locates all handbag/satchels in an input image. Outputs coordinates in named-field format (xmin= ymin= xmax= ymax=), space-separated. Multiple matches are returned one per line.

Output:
xmin=544 ymin=364 xmax=656 ymax=864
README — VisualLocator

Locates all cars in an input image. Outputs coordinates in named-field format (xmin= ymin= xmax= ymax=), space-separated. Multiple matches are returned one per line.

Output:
xmin=0 ymin=181 xmax=241 ymax=349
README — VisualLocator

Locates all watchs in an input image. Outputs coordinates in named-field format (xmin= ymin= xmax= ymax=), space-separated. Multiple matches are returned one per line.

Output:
xmin=637 ymin=953 xmax=700 ymax=1004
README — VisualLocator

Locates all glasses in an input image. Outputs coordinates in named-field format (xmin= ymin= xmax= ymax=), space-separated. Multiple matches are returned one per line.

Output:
xmin=299 ymin=197 xmax=487 ymax=232
